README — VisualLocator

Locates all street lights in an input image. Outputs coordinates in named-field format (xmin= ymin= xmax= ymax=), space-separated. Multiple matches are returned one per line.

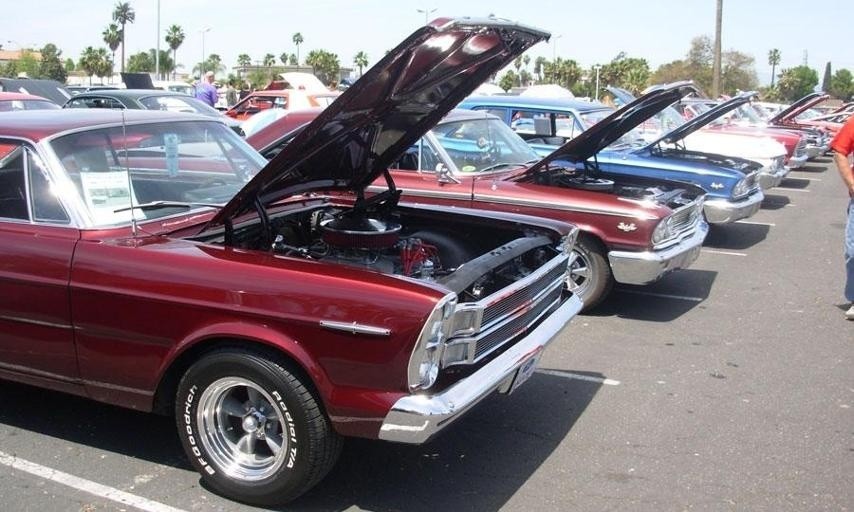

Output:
xmin=417 ymin=7 xmax=437 ymax=27
xmin=7 ymin=39 xmax=37 ymax=57
xmin=194 ymin=26 xmax=211 ymax=77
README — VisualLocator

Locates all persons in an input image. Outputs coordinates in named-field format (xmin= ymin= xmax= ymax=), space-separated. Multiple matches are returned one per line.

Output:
xmin=828 ymin=111 xmax=853 ymax=320
xmin=182 ymin=69 xmax=261 ymax=108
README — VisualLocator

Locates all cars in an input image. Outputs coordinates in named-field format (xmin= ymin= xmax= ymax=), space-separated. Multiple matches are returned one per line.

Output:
xmin=0 ymin=16 xmax=590 ymax=508
xmin=100 ymin=79 xmax=711 ymax=310
